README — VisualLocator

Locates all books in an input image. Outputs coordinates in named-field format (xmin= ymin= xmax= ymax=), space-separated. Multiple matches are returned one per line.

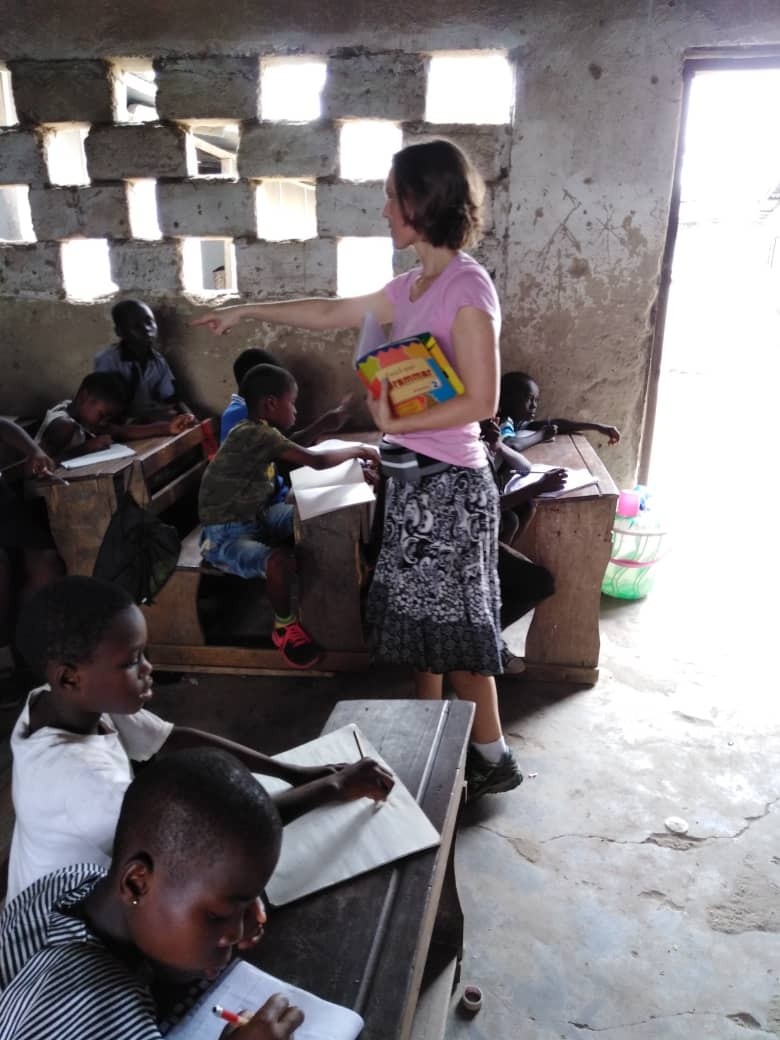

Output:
xmin=165 ymin=958 xmax=364 ymax=1040
xmin=252 ymin=723 xmax=440 ymax=906
xmin=353 ymin=309 xmax=465 ymax=420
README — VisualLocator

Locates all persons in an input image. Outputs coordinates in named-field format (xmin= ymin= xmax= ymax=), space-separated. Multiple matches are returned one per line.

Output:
xmin=186 ymin=137 xmax=522 ymax=801
xmin=197 ymin=364 xmax=382 ymax=668
xmin=478 ymin=418 xmax=569 ymax=674
xmin=221 ymin=348 xmax=366 ymax=506
xmin=31 ymin=372 xmax=196 ymax=465
xmin=0 ymin=417 xmax=58 ymax=644
xmin=6 ymin=574 xmax=394 ymax=920
xmin=0 ymin=746 xmax=304 ymax=1040
xmin=93 ymin=300 xmax=198 ymax=424
xmin=497 ymin=372 xmax=620 ymax=453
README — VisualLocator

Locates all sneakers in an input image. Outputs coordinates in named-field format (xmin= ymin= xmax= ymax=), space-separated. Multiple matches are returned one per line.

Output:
xmin=499 ymin=648 xmax=526 ymax=674
xmin=464 ymin=746 xmax=522 ymax=800
xmin=272 ymin=616 xmax=317 ymax=669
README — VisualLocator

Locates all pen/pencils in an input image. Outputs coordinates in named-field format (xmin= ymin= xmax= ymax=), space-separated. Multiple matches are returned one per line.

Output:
xmin=354 ymin=731 xmax=365 ymax=760
xmin=80 ymin=424 xmax=96 ymax=438
xmin=212 ymin=1005 xmax=251 ymax=1024
xmin=44 ymin=470 xmax=71 ymax=486
xmin=545 ymin=416 xmax=550 ymax=425
xmin=510 ymin=469 xmax=547 ymax=474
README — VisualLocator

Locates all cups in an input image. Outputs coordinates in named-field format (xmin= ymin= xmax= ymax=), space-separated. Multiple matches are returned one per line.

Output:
xmin=618 ymin=488 xmax=640 ymax=518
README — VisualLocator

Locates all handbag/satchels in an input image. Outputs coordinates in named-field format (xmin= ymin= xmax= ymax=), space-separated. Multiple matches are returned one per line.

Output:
xmin=91 ymin=493 xmax=182 ymax=604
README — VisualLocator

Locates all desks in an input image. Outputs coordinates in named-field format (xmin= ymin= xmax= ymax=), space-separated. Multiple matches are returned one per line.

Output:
xmin=172 ymin=699 xmax=477 ymax=1040
xmin=41 ymin=413 xmax=620 ymax=685
